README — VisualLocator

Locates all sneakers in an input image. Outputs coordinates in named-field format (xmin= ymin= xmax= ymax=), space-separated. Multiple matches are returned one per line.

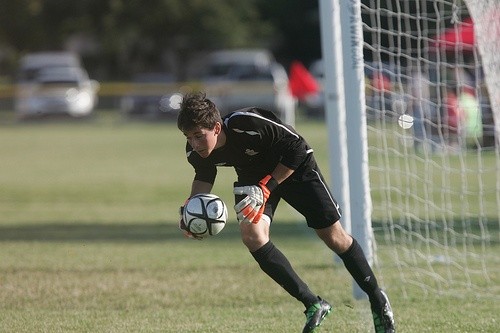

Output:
xmin=303 ymin=295 xmax=333 ymax=333
xmin=368 ymin=289 xmax=396 ymax=333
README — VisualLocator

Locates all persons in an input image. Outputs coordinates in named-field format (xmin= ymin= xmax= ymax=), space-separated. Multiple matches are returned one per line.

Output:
xmin=370 ymin=60 xmax=485 ymax=156
xmin=177 ymin=91 xmax=396 ymax=333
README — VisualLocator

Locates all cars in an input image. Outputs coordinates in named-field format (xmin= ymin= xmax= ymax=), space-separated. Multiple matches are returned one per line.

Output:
xmin=14 ymin=52 xmax=101 ymax=121
xmin=122 ymin=50 xmax=499 ymax=147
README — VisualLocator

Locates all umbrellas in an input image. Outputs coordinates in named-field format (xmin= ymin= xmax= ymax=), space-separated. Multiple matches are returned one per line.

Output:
xmin=430 ymin=12 xmax=477 ymax=51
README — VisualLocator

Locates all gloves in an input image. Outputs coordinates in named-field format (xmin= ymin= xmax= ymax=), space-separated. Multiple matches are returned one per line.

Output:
xmin=178 ymin=199 xmax=202 ymax=241
xmin=233 ymin=175 xmax=280 ymax=224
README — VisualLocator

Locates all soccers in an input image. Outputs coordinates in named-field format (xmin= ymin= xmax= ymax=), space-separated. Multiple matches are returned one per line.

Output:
xmin=182 ymin=193 xmax=228 ymax=239
